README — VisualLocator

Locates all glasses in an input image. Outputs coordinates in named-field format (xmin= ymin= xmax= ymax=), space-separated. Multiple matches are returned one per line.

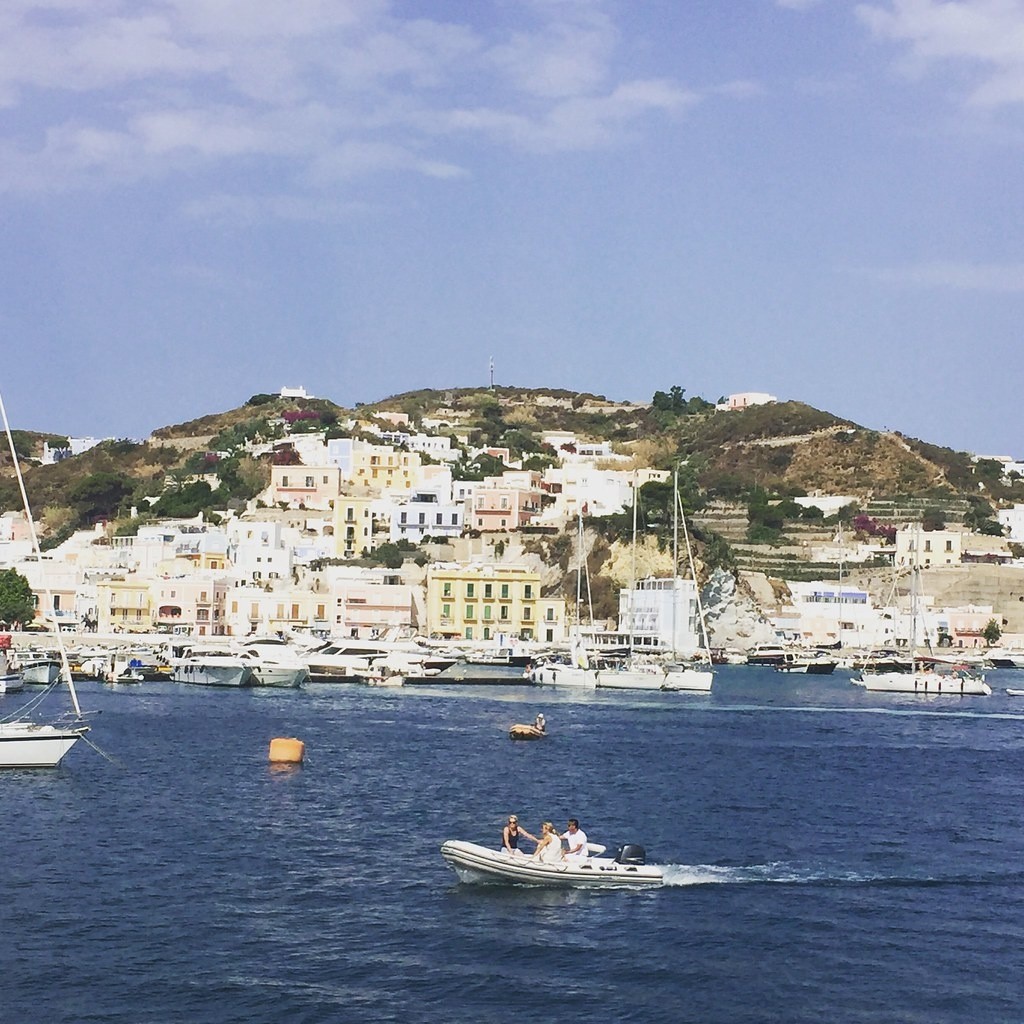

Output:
xmin=509 ymin=821 xmax=517 ymax=824
xmin=568 ymin=825 xmax=570 ymax=827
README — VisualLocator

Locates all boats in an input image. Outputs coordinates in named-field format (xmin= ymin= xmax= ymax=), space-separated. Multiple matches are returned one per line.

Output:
xmin=508 ymin=724 xmax=546 ymax=741
xmin=1 ymin=455 xmax=746 ymax=695
xmin=440 ymin=838 xmax=664 ymax=888
xmin=851 ymin=657 xmax=934 ymax=671
xmin=747 ymin=643 xmax=794 ymax=667
xmin=0 ymin=714 xmax=91 ymax=767
xmin=984 ymin=657 xmax=1024 ymax=669
xmin=857 ymin=541 xmax=991 ymax=696
xmin=267 ymin=738 xmax=305 ymax=763
xmin=915 ymin=655 xmax=983 ymax=668
xmin=773 ymin=661 xmax=839 ymax=675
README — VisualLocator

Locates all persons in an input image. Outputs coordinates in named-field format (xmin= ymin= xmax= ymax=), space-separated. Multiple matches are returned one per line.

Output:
xmin=501 ymin=814 xmax=543 ymax=857
xmin=558 ymin=818 xmax=589 ymax=862
xmin=534 ymin=712 xmax=546 ymax=733
xmin=529 ymin=822 xmax=563 ymax=864
